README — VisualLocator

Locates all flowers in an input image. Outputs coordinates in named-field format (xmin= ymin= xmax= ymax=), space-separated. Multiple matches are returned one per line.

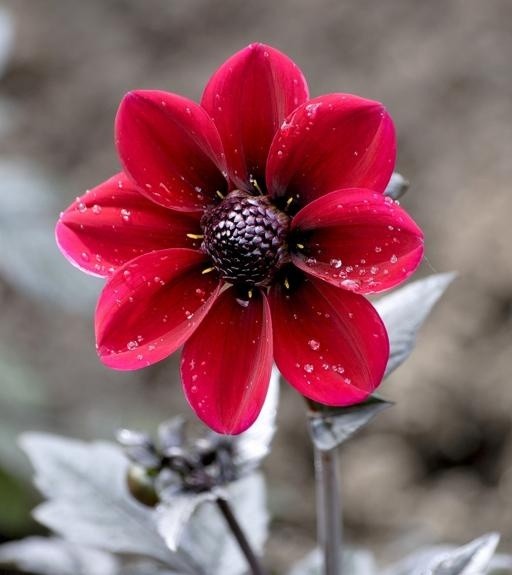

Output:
xmin=0 ymin=39 xmax=512 ymax=574
